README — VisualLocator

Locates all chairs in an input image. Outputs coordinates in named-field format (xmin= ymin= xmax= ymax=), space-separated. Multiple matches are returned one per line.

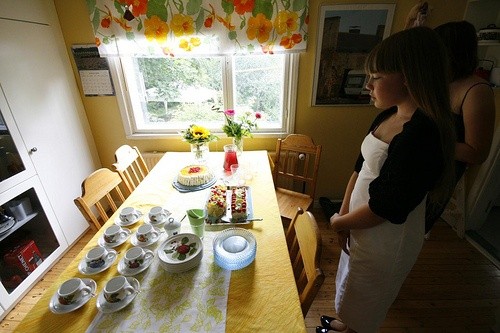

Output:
xmin=74 ymin=168 xmax=133 ymax=235
xmin=285 ymin=207 xmax=325 ymax=319
xmin=111 ymin=145 xmax=149 ymax=196
xmin=273 ymin=133 xmax=322 ymax=220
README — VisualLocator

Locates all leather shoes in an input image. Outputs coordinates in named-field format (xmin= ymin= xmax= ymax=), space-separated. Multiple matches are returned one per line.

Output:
xmin=316 ymin=315 xmax=339 ymax=333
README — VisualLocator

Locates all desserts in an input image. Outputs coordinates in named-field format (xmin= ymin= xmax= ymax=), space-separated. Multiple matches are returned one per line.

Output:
xmin=207 ymin=185 xmax=248 ymax=220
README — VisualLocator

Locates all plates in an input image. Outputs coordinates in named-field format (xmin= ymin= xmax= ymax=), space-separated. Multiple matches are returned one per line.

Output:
xmin=49 ymin=278 xmax=97 ymax=314
xmin=213 ymin=228 xmax=257 ymax=271
xmin=96 ymin=277 xmax=140 ymax=314
xmin=0 ymin=218 xmax=18 ymax=237
xmin=78 ymin=210 xmax=172 ymax=277
xmin=157 ymin=233 xmax=204 ymax=272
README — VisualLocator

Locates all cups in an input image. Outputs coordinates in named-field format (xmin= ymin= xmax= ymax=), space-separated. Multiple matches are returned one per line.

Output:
xmin=149 ymin=206 xmax=167 ymax=223
xmin=186 ymin=209 xmax=206 ymax=226
xmin=136 ymin=224 xmax=158 ymax=244
xmin=224 ymin=144 xmax=241 ymax=180
xmin=190 ymin=223 xmax=204 ymax=241
xmin=9 ymin=197 xmax=33 ymax=221
xmin=120 ymin=207 xmax=139 ymax=224
xmin=58 ymin=278 xmax=92 ymax=308
xmin=103 ymin=276 xmax=135 ymax=304
xmin=85 ymin=247 xmax=117 ymax=271
xmin=124 ymin=247 xmax=154 ymax=270
xmin=103 ymin=225 xmax=128 ymax=245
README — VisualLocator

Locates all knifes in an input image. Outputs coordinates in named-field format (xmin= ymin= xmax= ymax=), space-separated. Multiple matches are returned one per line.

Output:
xmin=221 ymin=217 xmax=262 ymax=222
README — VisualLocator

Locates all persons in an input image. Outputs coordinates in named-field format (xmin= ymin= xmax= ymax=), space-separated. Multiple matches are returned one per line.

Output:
xmin=314 ymin=27 xmax=450 ymax=333
xmin=425 ymin=21 xmax=495 ymax=236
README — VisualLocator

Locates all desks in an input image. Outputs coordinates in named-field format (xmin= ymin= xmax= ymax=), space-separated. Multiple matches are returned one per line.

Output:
xmin=13 ymin=152 xmax=306 ymax=333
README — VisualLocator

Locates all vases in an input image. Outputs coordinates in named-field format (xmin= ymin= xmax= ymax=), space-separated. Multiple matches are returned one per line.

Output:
xmin=232 ymin=138 xmax=243 ymax=155
xmin=190 ymin=142 xmax=209 ymax=164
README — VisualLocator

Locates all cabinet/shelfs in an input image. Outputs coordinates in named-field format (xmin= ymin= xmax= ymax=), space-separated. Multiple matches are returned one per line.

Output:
xmin=0 ymin=0 xmax=111 ymax=322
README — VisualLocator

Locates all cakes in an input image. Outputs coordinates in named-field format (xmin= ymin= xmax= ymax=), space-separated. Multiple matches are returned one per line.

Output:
xmin=177 ymin=165 xmax=212 ymax=186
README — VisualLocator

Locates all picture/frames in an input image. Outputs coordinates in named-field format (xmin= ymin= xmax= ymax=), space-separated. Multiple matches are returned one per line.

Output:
xmin=311 ymin=2 xmax=397 ymax=107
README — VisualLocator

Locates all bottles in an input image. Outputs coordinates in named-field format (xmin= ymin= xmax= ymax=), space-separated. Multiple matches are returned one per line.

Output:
xmin=164 ymin=218 xmax=181 ymax=238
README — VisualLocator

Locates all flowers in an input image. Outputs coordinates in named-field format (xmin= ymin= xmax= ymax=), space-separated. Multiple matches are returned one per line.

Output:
xmin=222 ymin=109 xmax=261 ymax=145
xmin=181 ymin=124 xmax=219 ymax=150
